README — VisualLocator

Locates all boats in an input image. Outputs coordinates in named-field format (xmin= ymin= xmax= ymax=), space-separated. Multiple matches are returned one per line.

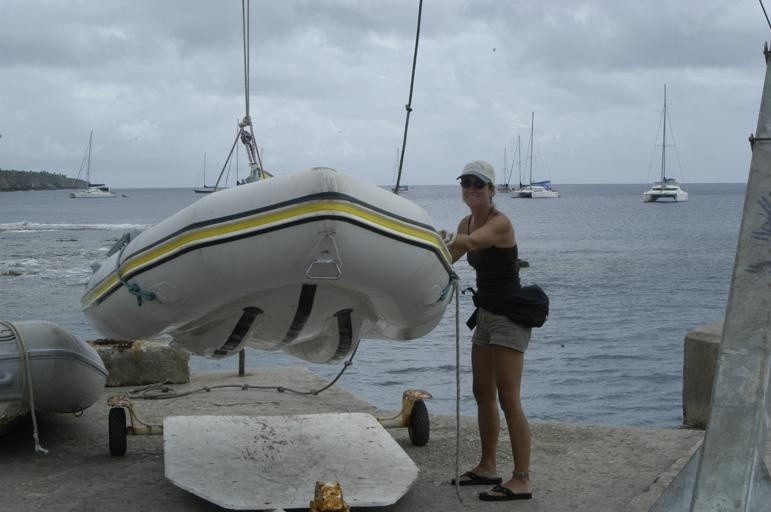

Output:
xmin=1 ymin=320 xmax=108 ymax=419
xmin=82 ymin=169 xmax=456 ymax=366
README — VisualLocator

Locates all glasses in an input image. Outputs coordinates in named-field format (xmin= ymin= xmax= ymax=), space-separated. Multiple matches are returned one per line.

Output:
xmin=460 ymin=178 xmax=485 ymax=190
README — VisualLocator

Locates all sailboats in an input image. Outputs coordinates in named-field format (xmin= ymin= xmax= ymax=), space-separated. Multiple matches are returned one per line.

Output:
xmin=392 ymin=145 xmax=409 ymax=191
xmin=496 ymin=144 xmax=515 ymax=193
xmin=71 ymin=129 xmax=117 ymax=199
xmin=509 ymin=108 xmax=560 ymax=200
xmin=193 ymin=153 xmax=224 ymax=194
xmin=644 ymin=83 xmax=690 ymax=202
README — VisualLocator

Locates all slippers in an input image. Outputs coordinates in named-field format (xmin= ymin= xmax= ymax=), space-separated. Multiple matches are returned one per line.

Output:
xmin=480 ymin=486 xmax=536 ymax=502
xmin=452 ymin=471 xmax=504 ymax=487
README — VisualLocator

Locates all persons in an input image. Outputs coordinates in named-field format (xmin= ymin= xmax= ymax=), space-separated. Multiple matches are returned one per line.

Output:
xmin=442 ymin=157 xmax=549 ymax=502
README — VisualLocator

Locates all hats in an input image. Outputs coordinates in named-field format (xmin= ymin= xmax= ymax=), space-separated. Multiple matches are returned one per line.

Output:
xmin=458 ymin=160 xmax=497 ymax=188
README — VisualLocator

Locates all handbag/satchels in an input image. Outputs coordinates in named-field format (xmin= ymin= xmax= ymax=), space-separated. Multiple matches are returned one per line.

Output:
xmin=475 ymin=286 xmax=549 ymax=328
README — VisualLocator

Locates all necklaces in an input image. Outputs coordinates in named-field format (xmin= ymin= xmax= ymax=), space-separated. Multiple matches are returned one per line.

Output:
xmin=468 ymin=207 xmax=494 ymax=238
